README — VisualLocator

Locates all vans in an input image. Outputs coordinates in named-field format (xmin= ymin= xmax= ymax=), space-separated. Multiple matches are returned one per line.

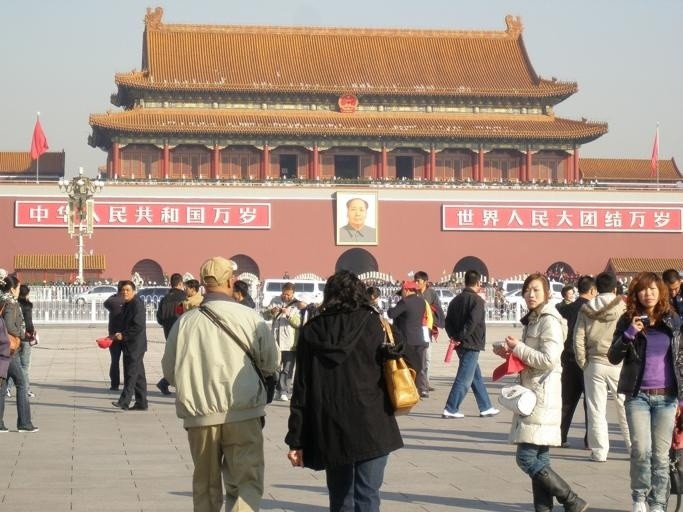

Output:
xmin=501 ymin=280 xmax=573 ymax=295
xmin=259 ymin=278 xmax=327 ymax=310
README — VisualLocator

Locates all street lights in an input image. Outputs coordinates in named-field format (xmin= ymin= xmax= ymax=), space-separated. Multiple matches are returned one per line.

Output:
xmin=56 ymin=165 xmax=106 ymax=285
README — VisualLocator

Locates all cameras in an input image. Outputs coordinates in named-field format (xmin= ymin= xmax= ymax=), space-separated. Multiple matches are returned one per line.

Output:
xmin=638 ymin=316 xmax=649 ymax=326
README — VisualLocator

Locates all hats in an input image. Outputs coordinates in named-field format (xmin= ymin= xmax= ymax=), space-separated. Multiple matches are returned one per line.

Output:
xmin=200 ymin=256 xmax=237 ymax=286
xmin=96 ymin=336 xmax=115 ymax=348
xmin=396 ymin=281 xmax=417 ymax=296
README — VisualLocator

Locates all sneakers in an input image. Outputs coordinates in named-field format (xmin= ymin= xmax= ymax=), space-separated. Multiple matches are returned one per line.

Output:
xmin=480 ymin=407 xmax=500 ymax=416
xmin=18 ymin=427 xmax=39 ymax=432
xmin=633 ymin=501 xmax=665 ymax=512
xmin=442 ymin=409 xmax=464 ymax=418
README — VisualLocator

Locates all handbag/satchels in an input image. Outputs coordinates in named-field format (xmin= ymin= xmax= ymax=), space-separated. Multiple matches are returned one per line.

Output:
xmin=384 ymin=357 xmax=420 ymax=416
xmin=498 ymin=383 xmax=537 ymax=416
xmin=8 ymin=333 xmax=20 ymax=353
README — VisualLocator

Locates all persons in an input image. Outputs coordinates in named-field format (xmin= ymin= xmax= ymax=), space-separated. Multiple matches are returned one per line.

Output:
xmin=492 ymin=269 xmax=682 ymax=512
xmin=95 ymin=281 xmax=149 ymax=410
xmin=339 ymin=198 xmax=376 ymax=243
xmin=1 ymin=276 xmax=39 ymax=433
xmin=157 ymin=257 xmax=500 ymax=512
xmin=41 ymin=279 xmax=157 ymax=300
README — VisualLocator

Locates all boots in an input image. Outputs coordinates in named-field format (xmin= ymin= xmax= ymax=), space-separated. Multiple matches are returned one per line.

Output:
xmin=532 ymin=466 xmax=588 ymax=512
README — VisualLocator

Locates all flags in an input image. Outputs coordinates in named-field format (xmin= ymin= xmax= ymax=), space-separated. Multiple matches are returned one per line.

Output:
xmin=650 ymin=129 xmax=658 ymax=177
xmin=30 ymin=120 xmax=49 ymax=160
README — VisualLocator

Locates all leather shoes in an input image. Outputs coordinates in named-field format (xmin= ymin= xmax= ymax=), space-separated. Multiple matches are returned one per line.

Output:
xmin=112 ymin=402 xmax=148 ymax=410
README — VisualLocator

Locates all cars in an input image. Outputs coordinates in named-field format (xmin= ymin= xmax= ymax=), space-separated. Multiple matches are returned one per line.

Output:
xmin=69 ymin=283 xmax=118 ymax=307
xmin=433 ymin=288 xmax=459 ymax=304
xmin=504 ymin=287 xmax=563 ymax=313
xmin=136 ymin=285 xmax=172 ymax=312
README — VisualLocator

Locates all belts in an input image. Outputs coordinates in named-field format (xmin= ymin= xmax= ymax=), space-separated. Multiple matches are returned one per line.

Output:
xmin=642 ymin=388 xmax=672 ymax=395
